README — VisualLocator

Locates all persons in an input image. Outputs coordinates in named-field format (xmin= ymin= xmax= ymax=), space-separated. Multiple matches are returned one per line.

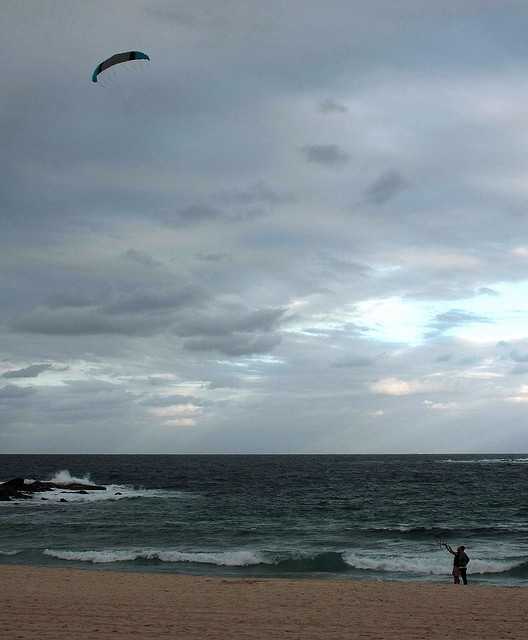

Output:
xmin=458 ymin=546 xmax=470 ymax=585
xmin=445 ymin=545 xmax=461 ymax=584
